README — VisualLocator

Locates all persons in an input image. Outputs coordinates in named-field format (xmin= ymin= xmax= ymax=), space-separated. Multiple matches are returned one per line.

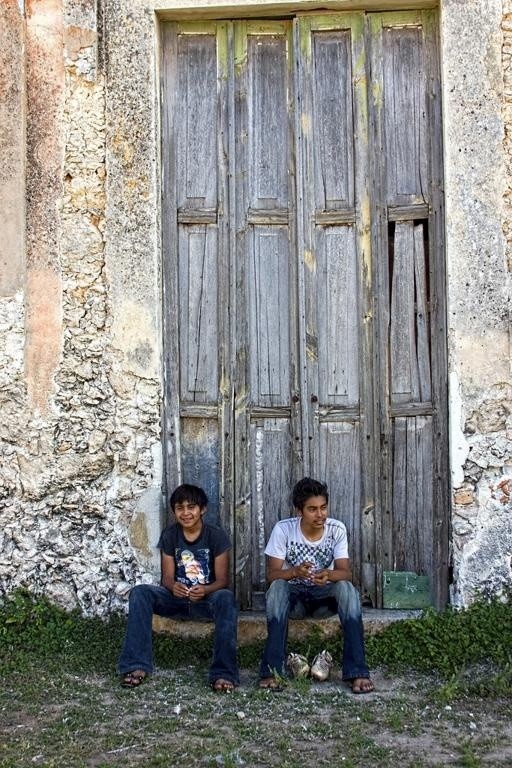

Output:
xmin=258 ymin=477 xmax=375 ymax=691
xmin=118 ymin=483 xmax=240 ymax=691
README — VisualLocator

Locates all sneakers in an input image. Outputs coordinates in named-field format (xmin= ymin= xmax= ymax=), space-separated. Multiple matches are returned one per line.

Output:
xmin=311 ymin=648 xmax=334 ymax=681
xmin=286 ymin=652 xmax=310 ymax=679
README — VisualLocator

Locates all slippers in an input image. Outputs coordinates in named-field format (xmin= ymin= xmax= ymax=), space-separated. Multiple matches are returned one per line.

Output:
xmin=122 ymin=672 xmax=144 ymax=688
